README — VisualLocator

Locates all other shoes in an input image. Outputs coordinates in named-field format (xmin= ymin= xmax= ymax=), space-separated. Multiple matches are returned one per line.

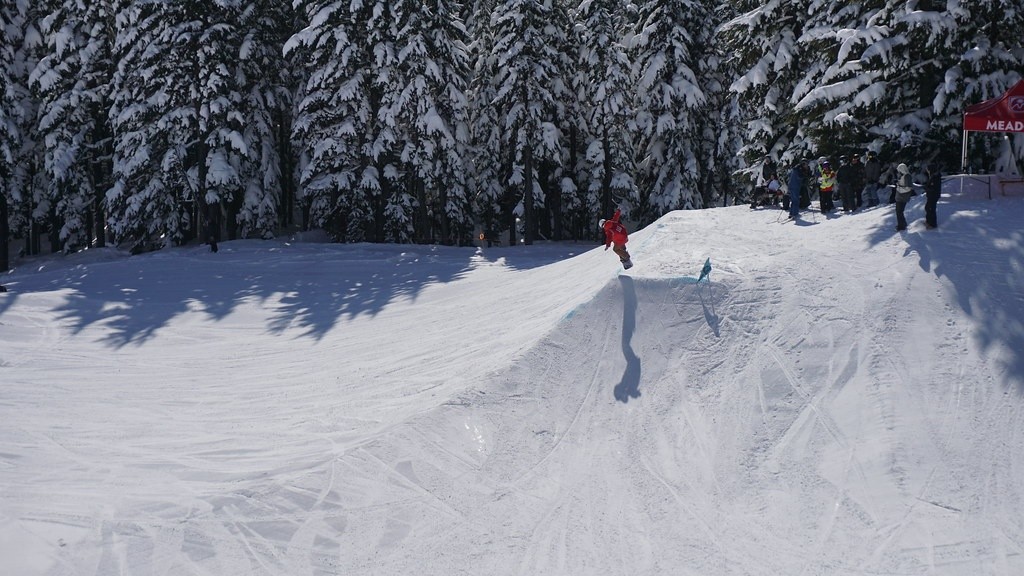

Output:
xmin=623 ymin=260 xmax=633 ymax=270
xmin=923 ymin=221 xmax=933 ymax=229
xmin=895 ymin=225 xmax=907 ymax=230
xmin=780 ymin=207 xmax=787 ymax=210
xmin=789 ymin=214 xmax=799 ymax=218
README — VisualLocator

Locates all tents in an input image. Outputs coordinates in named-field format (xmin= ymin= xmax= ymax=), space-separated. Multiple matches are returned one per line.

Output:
xmin=961 ymin=78 xmax=1024 ymax=171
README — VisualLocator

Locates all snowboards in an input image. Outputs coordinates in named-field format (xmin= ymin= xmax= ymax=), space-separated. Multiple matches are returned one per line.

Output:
xmin=620 ymin=246 xmax=633 ymax=270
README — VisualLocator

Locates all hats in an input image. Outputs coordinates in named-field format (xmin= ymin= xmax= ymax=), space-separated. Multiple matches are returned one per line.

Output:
xmin=868 ymin=151 xmax=877 ymax=156
xmin=840 ymin=155 xmax=848 ymax=158
xmin=801 ymin=158 xmax=808 ymax=161
xmin=599 ymin=219 xmax=606 ymax=227
xmin=823 ymin=161 xmax=829 ymax=164
xmin=765 ymin=155 xmax=771 ymax=158
xmin=853 ymin=154 xmax=859 ymax=157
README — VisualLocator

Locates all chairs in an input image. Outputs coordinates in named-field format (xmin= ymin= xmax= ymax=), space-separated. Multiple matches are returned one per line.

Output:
xmin=758 ymin=190 xmax=779 ymax=210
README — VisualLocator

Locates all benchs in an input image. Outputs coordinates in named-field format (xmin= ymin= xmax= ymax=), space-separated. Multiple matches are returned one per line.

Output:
xmin=999 ymin=180 xmax=1024 ymax=196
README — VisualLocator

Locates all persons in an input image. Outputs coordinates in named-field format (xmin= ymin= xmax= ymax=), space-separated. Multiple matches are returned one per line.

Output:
xmin=892 ymin=163 xmax=915 ymax=230
xmin=922 ymin=164 xmax=941 ymax=230
xmin=788 ymin=151 xmax=881 ymax=217
xmin=599 ymin=205 xmax=630 ymax=263
xmin=762 ymin=155 xmax=780 ymax=206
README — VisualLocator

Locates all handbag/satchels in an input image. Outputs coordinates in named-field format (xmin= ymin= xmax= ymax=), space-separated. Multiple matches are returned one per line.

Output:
xmin=909 ymin=189 xmax=917 ymax=197
xmin=768 ymin=180 xmax=780 ymax=191
xmin=896 ymin=174 xmax=912 ymax=193
